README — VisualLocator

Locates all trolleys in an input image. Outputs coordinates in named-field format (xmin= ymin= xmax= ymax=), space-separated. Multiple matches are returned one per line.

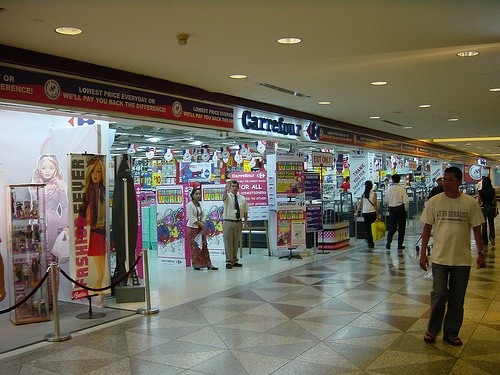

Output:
xmin=412 ymin=211 xmax=435 ymax=251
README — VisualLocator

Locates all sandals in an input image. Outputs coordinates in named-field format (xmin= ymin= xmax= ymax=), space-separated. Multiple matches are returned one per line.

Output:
xmin=443 ymin=336 xmax=463 ymax=346
xmin=424 ymin=331 xmax=434 ymax=343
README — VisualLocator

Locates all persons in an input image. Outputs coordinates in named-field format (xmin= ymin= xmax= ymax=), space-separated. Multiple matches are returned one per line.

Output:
xmin=416 ymin=177 xmax=427 ymax=189
xmin=293 ymin=234 xmax=301 ymax=242
xmin=13 ymin=199 xmax=50 ymax=317
xmin=354 ymin=180 xmax=379 ymax=249
xmin=341 ymin=177 xmax=350 ymax=193
xmin=32 ymin=155 xmax=68 ymax=312
xmin=426 ymin=178 xmax=438 ymax=191
xmin=478 ymin=177 xmax=497 ymax=247
xmin=477 ymin=176 xmax=485 ymax=203
xmin=428 ymin=178 xmax=445 ymax=199
xmin=381 ymin=178 xmax=389 ymax=189
xmin=383 ymin=174 xmax=408 ymax=250
xmin=221 ymin=181 xmax=250 ymax=269
xmin=419 ymin=166 xmax=486 ymax=347
xmin=186 ymin=189 xmax=219 ymax=271
xmin=0 ymin=239 xmax=6 ymax=302
xmin=73 ymin=157 xmax=109 ymax=295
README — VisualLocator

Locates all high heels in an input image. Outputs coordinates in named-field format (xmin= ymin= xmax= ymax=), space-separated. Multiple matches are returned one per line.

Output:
xmin=195 ymin=268 xmax=200 ymax=270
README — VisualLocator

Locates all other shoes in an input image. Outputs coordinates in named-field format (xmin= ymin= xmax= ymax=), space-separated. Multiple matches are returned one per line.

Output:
xmin=208 ymin=266 xmax=218 ymax=270
xmin=398 ymin=246 xmax=405 ymax=249
xmin=386 ymin=241 xmax=390 ymax=249
xmin=490 ymin=239 xmax=495 ymax=246
xmin=226 ymin=264 xmax=232 ymax=269
xmin=233 ymin=263 xmax=242 ymax=267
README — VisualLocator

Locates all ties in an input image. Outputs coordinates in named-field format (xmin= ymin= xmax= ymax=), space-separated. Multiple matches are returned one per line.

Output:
xmin=235 ymin=194 xmax=240 ymax=220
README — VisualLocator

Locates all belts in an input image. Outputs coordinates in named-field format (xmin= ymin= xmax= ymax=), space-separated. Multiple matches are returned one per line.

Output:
xmin=224 ymin=220 xmax=242 ymax=222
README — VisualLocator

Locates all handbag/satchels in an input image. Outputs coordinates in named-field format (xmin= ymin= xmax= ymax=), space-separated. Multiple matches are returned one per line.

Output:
xmin=494 ymin=207 xmax=499 ymax=218
xmin=371 ymin=219 xmax=385 ymax=240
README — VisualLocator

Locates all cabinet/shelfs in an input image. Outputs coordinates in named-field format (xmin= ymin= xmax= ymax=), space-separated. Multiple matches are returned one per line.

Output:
xmin=317 ymin=200 xmax=349 ymax=250
xmin=5 ymin=183 xmax=52 ymax=325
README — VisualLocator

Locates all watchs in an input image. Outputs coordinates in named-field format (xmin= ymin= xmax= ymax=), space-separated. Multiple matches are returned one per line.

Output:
xmin=478 ymin=250 xmax=486 ymax=256
